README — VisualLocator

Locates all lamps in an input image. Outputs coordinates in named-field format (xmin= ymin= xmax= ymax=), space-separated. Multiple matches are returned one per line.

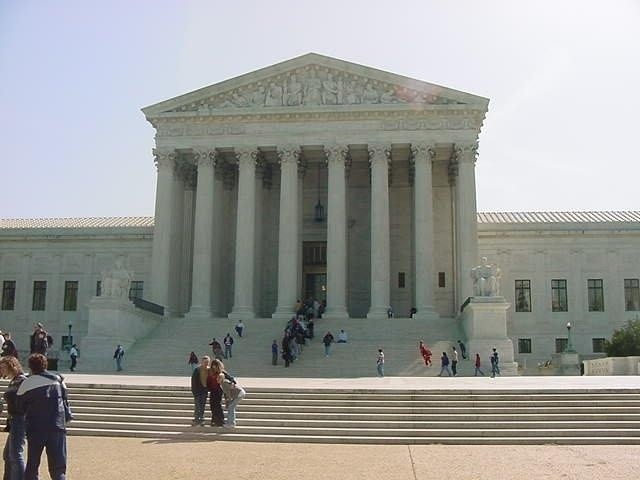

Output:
xmin=315 ymin=163 xmax=324 ymax=222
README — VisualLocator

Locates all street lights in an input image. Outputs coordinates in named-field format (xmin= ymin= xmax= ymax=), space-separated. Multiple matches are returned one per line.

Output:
xmin=565 ymin=322 xmax=573 ymax=348
xmin=67 ymin=320 xmax=73 ymax=345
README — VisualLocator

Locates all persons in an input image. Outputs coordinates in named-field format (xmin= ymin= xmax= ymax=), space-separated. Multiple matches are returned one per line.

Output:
xmin=336 ymin=329 xmax=349 ymax=344
xmin=14 ymin=353 xmax=76 ymax=480
xmin=270 ymin=296 xmax=327 ymax=369
xmin=113 ymin=345 xmax=125 ymax=372
xmin=376 ymin=348 xmax=386 ymax=378
xmin=0 ymin=321 xmax=50 ymax=433
xmin=323 ymin=331 xmax=334 ymax=358
xmin=208 ymin=358 xmax=236 ymax=428
xmin=69 ymin=343 xmax=79 ymax=372
xmin=209 ymin=337 xmax=224 ymax=356
xmin=224 ymin=332 xmax=234 ymax=360
xmin=0 ymin=356 xmax=30 ymax=480
xmin=409 ymin=306 xmax=417 ymax=318
xmin=234 ymin=319 xmax=245 ymax=337
xmin=419 ymin=339 xmax=501 ymax=379
xmin=215 ymin=343 xmax=223 ymax=362
xmin=188 ymin=351 xmax=200 ymax=372
xmin=213 ymin=68 xmax=408 ymax=109
xmin=387 ymin=303 xmax=394 ymax=320
xmin=473 ymin=255 xmax=498 ymax=297
xmin=215 ymin=372 xmax=246 ymax=428
xmin=190 ymin=355 xmax=212 ymax=427
xmin=101 ymin=256 xmax=130 ymax=297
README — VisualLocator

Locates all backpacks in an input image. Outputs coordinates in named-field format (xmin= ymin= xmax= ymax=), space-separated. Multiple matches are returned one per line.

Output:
xmin=46 ymin=334 xmax=53 ymax=348
